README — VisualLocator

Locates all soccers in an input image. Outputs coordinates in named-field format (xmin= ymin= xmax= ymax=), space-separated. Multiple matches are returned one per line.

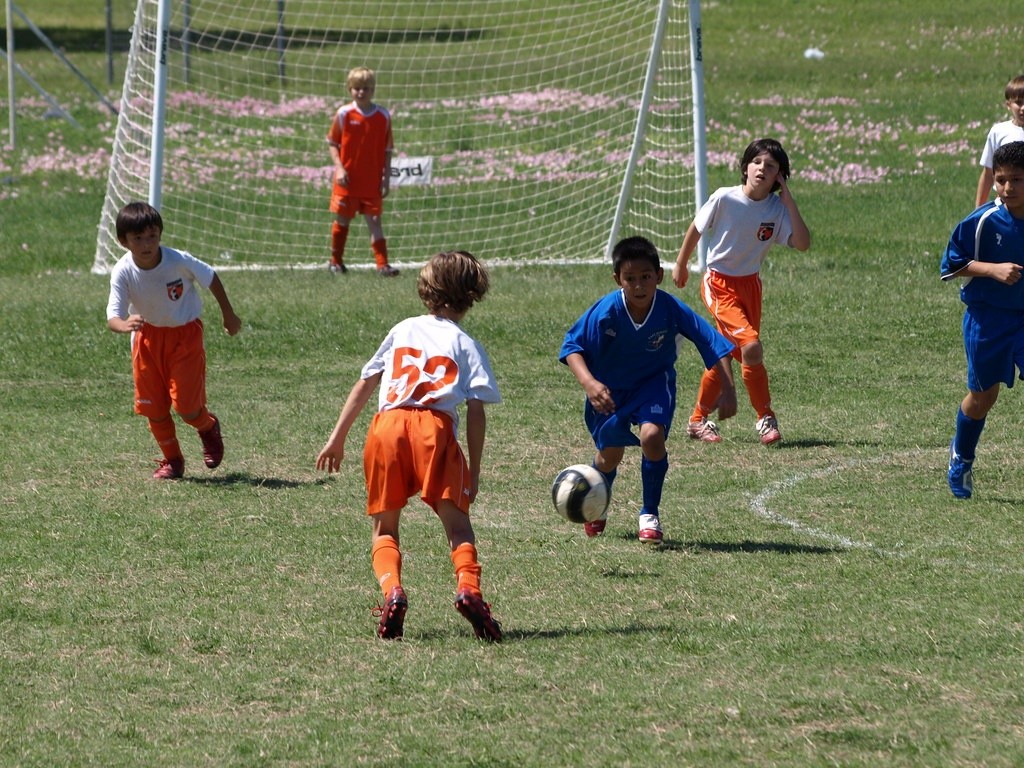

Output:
xmin=552 ymin=463 xmax=612 ymax=524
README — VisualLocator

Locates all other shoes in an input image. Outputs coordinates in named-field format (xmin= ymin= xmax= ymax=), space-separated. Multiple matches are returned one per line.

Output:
xmin=330 ymin=264 xmax=345 ymax=274
xmin=379 ymin=264 xmax=400 ymax=277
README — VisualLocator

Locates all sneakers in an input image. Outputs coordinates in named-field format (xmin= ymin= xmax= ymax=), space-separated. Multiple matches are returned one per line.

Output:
xmin=152 ymin=455 xmax=185 ymax=478
xmin=639 ymin=514 xmax=663 ymax=543
xmin=196 ymin=412 xmax=224 ymax=468
xmin=686 ymin=416 xmax=720 ymax=443
xmin=756 ymin=412 xmax=781 ymax=444
xmin=372 ymin=586 xmax=408 ymax=641
xmin=454 ymin=589 xmax=503 ymax=643
xmin=585 ymin=512 xmax=607 ymax=537
xmin=948 ymin=438 xmax=976 ymax=498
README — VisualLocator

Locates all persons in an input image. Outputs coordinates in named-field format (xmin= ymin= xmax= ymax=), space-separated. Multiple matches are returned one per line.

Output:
xmin=556 ymin=234 xmax=735 ymax=542
xmin=106 ymin=201 xmax=242 ymax=479
xmin=672 ymin=139 xmax=811 ymax=445
xmin=324 ymin=66 xmax=401 ymax=277
xmin=940 ymin=143 xmax=1024 ymax=500
xmin=975 ymin=73 xmax=1024 ymax=209
xmin=313 ymin=248 xmax=500 ymax=642
